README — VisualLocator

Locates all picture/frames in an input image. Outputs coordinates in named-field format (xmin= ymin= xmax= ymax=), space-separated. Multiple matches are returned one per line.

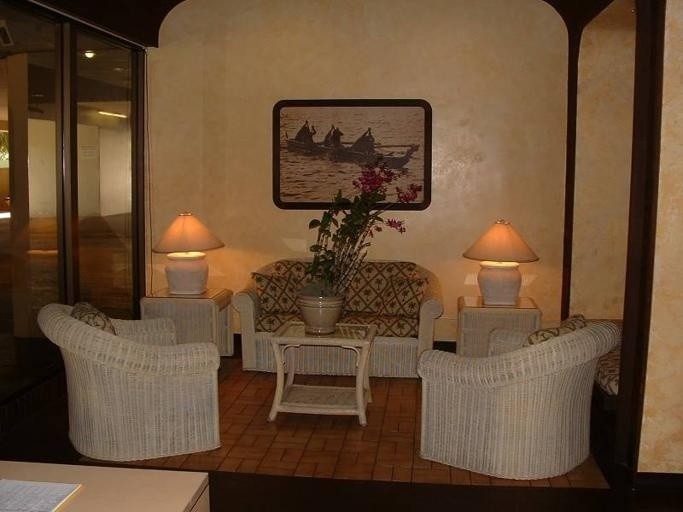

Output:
xmin=271 ymin=99 xmax=432 ymax=210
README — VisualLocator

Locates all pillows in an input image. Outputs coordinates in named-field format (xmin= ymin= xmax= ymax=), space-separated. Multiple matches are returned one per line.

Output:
xmin=249 ymin=269 xmax=300 ymax=316
xmin=375 ymin=277 xmax=428 ymax=319
xmin=521 ymin=313 xmax=584 ymax=348
xmin=69 ymin=299 xmax=114 ymax=335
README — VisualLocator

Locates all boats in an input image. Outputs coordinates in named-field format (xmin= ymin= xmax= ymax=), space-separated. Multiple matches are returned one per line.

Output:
xmin=284 ymin=137 xmax=418 ymax=169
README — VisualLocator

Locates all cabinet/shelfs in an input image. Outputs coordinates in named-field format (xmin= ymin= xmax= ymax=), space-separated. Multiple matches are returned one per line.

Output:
xmin=453 ymin=291 xmax=543 ymax=353
xmin=141 ymin=290 xmax=232 ymax=356
xmin=0 ymin=453 xmax=214 ymax=511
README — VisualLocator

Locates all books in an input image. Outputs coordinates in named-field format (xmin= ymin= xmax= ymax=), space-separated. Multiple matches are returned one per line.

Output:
xmin=0 ymin=479 xmax=82 ymax=512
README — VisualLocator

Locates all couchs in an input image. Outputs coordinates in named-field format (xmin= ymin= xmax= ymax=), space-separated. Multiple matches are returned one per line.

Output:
xmin=37 ymin=300 xmax=220 ymax=461
xmin=230 ymin=259 xmax=442 ymax=381
xmin=419 ymin=306 xmax=618 ymax=481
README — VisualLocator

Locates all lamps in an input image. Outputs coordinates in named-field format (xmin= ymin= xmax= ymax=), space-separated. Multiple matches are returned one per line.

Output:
xmin=151 ymin=211 xmax=225 ymax=294
xmin=460 ymin=216 xmax=541 ymax=306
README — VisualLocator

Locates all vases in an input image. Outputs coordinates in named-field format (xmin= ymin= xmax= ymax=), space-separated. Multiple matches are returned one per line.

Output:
xmin=294 ymin=298 xmax=342 ymax=336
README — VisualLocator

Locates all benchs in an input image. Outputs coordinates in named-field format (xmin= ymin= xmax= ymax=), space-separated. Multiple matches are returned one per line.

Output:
xmin=590 ymin=319 xmax=624 ymax=490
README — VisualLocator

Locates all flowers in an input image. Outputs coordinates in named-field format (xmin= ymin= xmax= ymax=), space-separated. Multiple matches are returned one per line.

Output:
xmin=304 ymin=162 xmax=422 ymax=298
